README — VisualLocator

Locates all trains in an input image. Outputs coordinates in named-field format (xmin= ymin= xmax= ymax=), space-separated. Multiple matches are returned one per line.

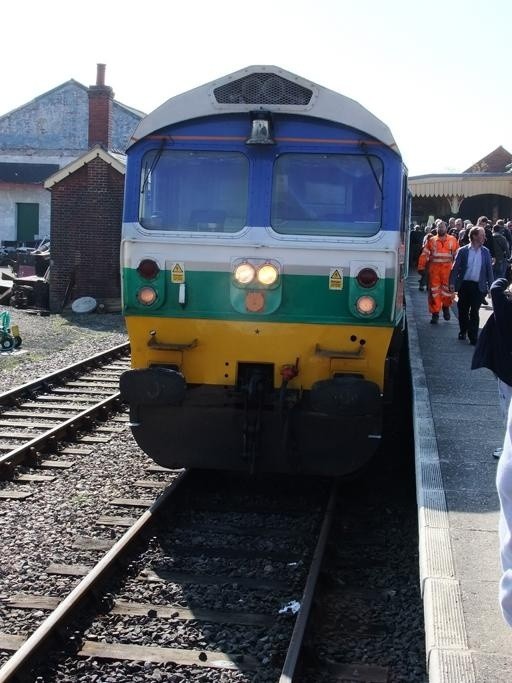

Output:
xmin=119 ymin=65 xmax=412 ymax=474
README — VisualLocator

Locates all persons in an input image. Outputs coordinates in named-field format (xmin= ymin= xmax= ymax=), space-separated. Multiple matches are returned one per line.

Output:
xmin=496 ymin=396 xmax=512 ymax=628
xmin=449 ymin=227 xmax=494 ymax=346
xmin=471 ymin=278 xmax=512 ymax=460
xmin=409 ymin=217 xmax=512 ymax=325
xmin=317 ymin=174 xmax=381 ymax=221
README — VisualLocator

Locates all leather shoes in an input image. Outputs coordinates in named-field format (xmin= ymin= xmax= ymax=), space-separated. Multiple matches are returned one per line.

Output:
xmin=430 ymin=304 xmax=478 ymax=344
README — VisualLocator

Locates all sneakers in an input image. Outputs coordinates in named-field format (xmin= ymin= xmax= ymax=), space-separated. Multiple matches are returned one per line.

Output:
xmin=493 ymin=448 xmax=503 ymax=458
